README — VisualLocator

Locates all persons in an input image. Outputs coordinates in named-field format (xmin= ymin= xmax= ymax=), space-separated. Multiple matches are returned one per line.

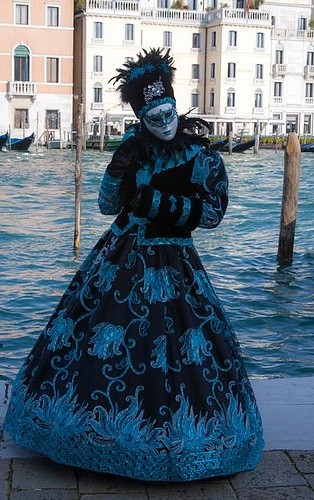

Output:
xmin=4 ymin=47 xmax=265 ymax=483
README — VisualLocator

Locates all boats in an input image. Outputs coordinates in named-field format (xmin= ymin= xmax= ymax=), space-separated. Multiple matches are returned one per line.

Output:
xmin=0 ymin=130 xmax=8 ymax=152
xmin=210 ymin=137 xmax=229 ymax=152
xmin=3 ymin=131 xmax=35 ymax=152
xmin=301 ymin=143 xmax=314 ymax=152
xmin=220 ymin=138 xmax=254 ymax=153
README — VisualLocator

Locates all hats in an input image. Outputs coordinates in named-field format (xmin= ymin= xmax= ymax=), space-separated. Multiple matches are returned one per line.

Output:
xmin=108 ymin=47 xmax=176 ymax=121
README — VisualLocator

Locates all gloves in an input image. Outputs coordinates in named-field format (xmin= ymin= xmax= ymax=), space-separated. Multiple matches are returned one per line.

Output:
xmin=107 ymin=136 xmax=134 ymax=179
xmin=118 ymin=185 xmax=144 ymax=214
